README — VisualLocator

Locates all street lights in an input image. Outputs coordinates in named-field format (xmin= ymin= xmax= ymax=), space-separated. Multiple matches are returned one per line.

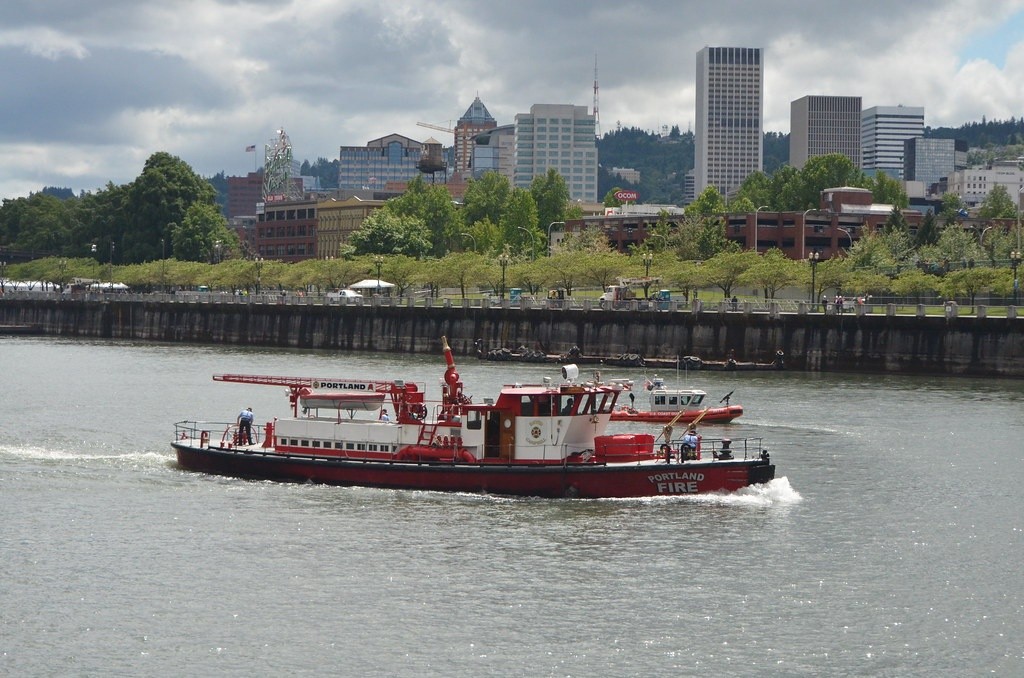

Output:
xmin=653 ymin=234 xmax=666 ymax=252
xmin=802 ymin=208 xmax=817 ymax=259
xmin=643 ymin=253 xmax=652 ymax=297
xmin=461 ymin=233 xmax=475 ymax=251
xmin=161 ymin=238 xmax=165 ymax=300
xmin=499 ymin=255 xmax=509 ymax=299
xmin=837 ymin=228 xmax=853 ymax=259
xmin=969 ymin=226 xmax=993 ymax=245
xmin=547 ymin=222 xmax=564 ymax=257
xmin=374 ymin=256 xmax=383 ymax=294
xmin=755 ymin=205 xmax=769 ymax=293
xmin=215 ymin=239 xmax=222 ymax=262
xmin=1011 ymin=250 xmax=1021 ymax=304
xmin=809 ymin=252 xmax=819 ymax=311
xmin=58 ymin=261 xmax=67 ymax=294
xmin=518 ymin=227 xmax=534 ymax=262
xmin=0 ymin=261 xmax=6 ymax=297
xmin=255 ymin=258 xmax=264 ymax=293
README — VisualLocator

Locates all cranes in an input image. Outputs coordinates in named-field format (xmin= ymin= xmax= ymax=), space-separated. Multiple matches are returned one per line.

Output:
xmin=416 ymin=119 xmax=475 ymax=171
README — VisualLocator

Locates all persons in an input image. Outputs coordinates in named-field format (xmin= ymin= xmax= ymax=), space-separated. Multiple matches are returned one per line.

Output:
xmin=834 ymin=296 xmax=843 ymax=314
xmin=282 ymin=290 xmax=286 ymax=304
xmin=296 ymin=290 xmax=300 ymax=304
xmin=561 ymin=398 xmax=573 ymax=413
xmin=382 ymin=409 xmax=389 ymax=421
xmin=683 ymin=431 xmax=698 ymax=459
xmin=237 ymin=408 xmax=256 ymax=446
xmin=236 ymin=289 xmax=243 ymax=302
xmin=822 ymin=295 xmax=828 ymax=314
xmin=732 ymin=295 xmax=737 ymax=311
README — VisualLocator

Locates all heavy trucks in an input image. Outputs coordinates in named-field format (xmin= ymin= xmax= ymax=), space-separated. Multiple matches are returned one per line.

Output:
xmin=599 ymin=286 xmax=663 ymax=310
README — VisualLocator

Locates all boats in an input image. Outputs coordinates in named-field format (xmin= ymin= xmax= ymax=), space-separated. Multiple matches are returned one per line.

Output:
xmin=171 ymin=335 xmax=775 ymax=499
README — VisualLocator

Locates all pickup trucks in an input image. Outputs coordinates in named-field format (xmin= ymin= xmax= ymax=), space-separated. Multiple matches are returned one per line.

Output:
xmin=327 ymin=290 xmax=363 ymax=303
